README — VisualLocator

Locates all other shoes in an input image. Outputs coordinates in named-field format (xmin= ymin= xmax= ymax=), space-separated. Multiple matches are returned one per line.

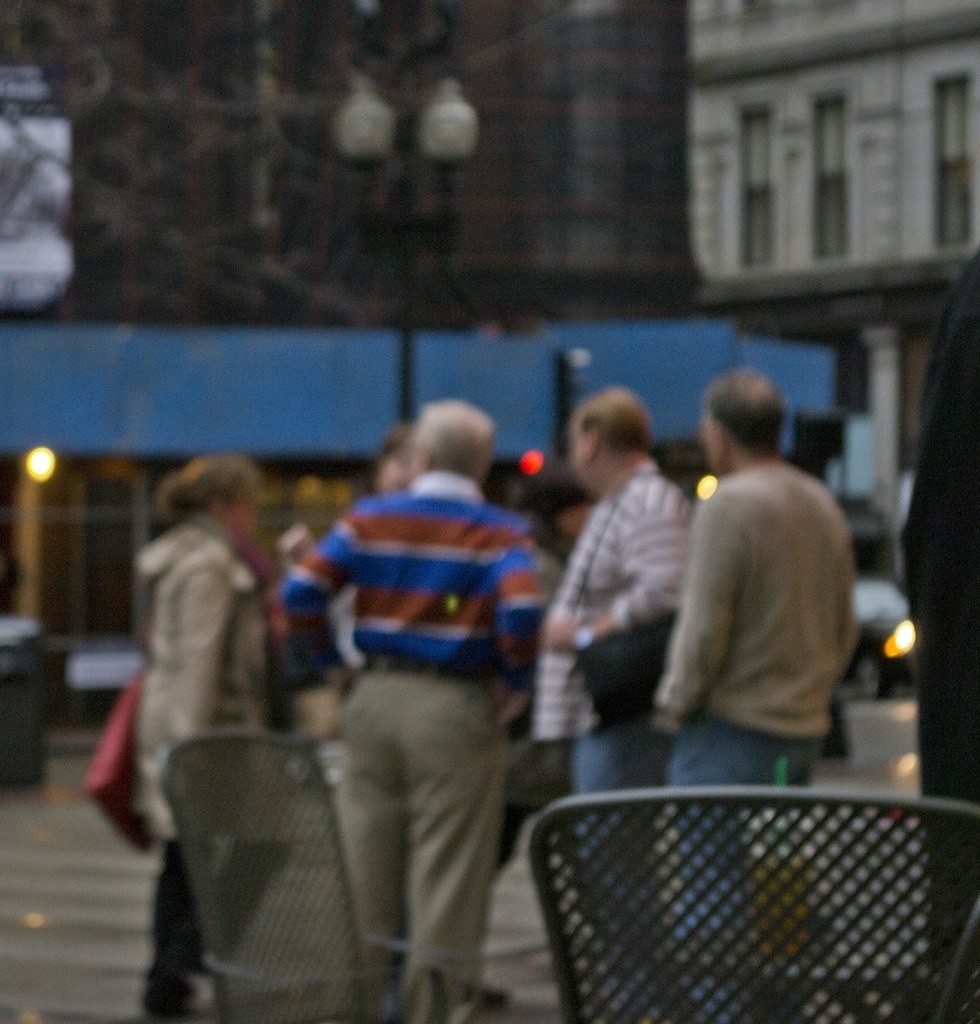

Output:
xmin=467 ymin=981 xmax=509 ymax=1008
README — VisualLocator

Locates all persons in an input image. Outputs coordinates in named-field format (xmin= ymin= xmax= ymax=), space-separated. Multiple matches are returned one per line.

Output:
xmin=532 ymin=385 xmax=696 ymax=1024
xmin=134 ymin=451 xmax=265 ymax=1024
xmin=897 ymin=246 xmax=980 ymax=984
xmin=281 ymin=397 xmax=566 ymax=1023
xmin=650 ymin=367 xmax=856 ymax=1024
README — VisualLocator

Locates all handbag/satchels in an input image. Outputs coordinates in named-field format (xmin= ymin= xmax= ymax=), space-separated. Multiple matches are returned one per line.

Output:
xmin=572 ymin=474 xmax=677 ymax=728
xmin=88 ymin=675 xmax=154 ymax=850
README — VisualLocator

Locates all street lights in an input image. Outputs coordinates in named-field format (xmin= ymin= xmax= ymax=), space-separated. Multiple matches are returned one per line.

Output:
xmin=326 ymin=69 xmax=480 ymax=423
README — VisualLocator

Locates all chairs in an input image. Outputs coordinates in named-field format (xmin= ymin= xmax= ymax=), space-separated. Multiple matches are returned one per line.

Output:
xmin=527 ymin=782 xmax=980 ymax=1024
xmin=168 ymin=731 xmax=377 ymax=1024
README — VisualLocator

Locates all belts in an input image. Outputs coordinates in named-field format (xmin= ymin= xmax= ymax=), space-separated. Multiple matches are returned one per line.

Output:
xmin=364 ymin=656 xmax=480 ymax=680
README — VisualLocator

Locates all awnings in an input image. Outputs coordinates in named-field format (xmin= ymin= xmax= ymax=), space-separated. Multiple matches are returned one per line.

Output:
xmin=410 ymin=336 xmax=562 ymax=463
xmin=545 ymin=314 xmax=840 ymax=452
xmin=0 ymin=322 xmax=404 ymax=469
